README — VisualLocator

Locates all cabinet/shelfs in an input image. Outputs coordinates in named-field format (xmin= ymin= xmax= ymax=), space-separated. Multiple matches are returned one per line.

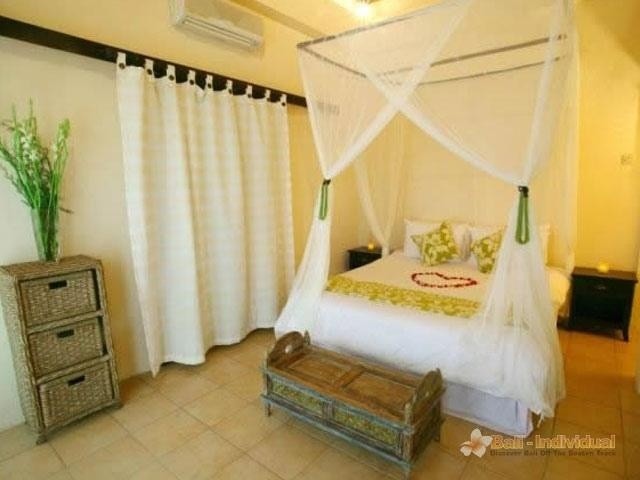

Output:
xmin=0 ymin=251 xmax=125 ymax=447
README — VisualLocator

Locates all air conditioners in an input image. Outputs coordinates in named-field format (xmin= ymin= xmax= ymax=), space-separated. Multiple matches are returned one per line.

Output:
xmin=166 ymin=0 xmax=267 ymax=54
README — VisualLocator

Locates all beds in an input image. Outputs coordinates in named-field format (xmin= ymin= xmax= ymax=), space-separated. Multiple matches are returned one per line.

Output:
xmin=271 ymin=245 xmax=573 ymax=446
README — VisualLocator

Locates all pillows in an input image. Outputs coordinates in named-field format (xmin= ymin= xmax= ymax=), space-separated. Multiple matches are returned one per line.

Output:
xmin=408 ymin=217 xmax=461 ymax=267
xmin=466 ymin=224 xmax=506 ymax=273
xmin=459 ymin=222 xmax=554 ymax=273
xmin=400 ymin=218 xmax=473 ymax=266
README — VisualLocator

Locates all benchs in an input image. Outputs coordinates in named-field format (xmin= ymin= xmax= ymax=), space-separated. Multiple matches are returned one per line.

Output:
xmin=254 ymin=326 xmax=448 ymax=478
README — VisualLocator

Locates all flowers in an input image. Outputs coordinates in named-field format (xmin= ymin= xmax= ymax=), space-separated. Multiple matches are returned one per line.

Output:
xmin=0 ymin=89 xmax=82 ymax=268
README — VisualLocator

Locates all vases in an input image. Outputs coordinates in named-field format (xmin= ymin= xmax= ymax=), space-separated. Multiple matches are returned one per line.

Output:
xmin=30 ymin=205 xmax=62 ymax=267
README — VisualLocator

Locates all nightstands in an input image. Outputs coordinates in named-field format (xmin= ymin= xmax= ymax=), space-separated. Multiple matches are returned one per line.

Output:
xmin=564 ymin=264 xmax=639 ymax=343
xmin=346 ymin=243 xmax=393 ymax=271
